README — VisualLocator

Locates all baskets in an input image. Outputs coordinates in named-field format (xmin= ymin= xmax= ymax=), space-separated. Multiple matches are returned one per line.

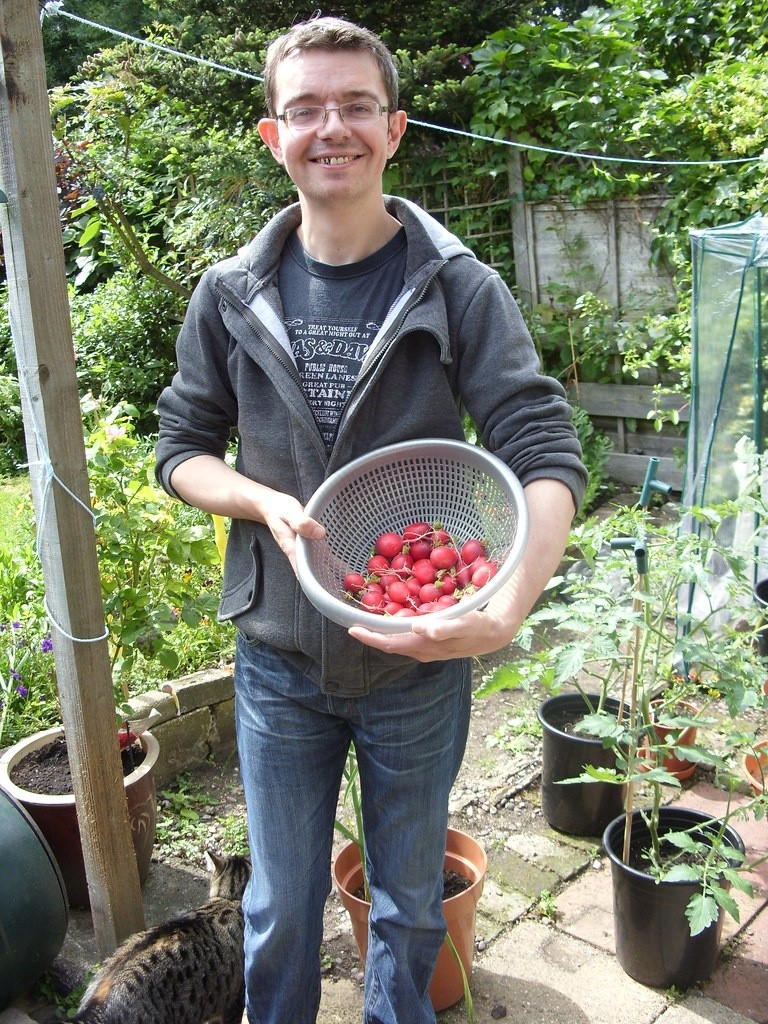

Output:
xmin=295 ymin=437 xmax=530 ymax=635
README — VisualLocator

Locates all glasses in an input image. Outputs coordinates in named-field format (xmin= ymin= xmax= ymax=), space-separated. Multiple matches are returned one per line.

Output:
xmin=277 ymin=101 xmax=394 ymax=131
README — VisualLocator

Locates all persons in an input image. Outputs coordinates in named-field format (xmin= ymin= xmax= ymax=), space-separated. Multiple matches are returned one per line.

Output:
xmin=154 ymin=17 xmax=588 ymax=1024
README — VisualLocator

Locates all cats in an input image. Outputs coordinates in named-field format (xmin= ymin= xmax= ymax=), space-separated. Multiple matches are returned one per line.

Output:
xmin=52 ymin=850 xmax=253 ymax=1024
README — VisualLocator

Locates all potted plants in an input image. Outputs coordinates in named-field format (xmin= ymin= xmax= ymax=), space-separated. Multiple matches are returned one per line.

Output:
xmin=531 ymin=504 xmax=768 ymax=989
xmin=0 ymin=393 xmax=218 ymax=909
xmin=321 ymin=739 xmax=488 ymax=1013
